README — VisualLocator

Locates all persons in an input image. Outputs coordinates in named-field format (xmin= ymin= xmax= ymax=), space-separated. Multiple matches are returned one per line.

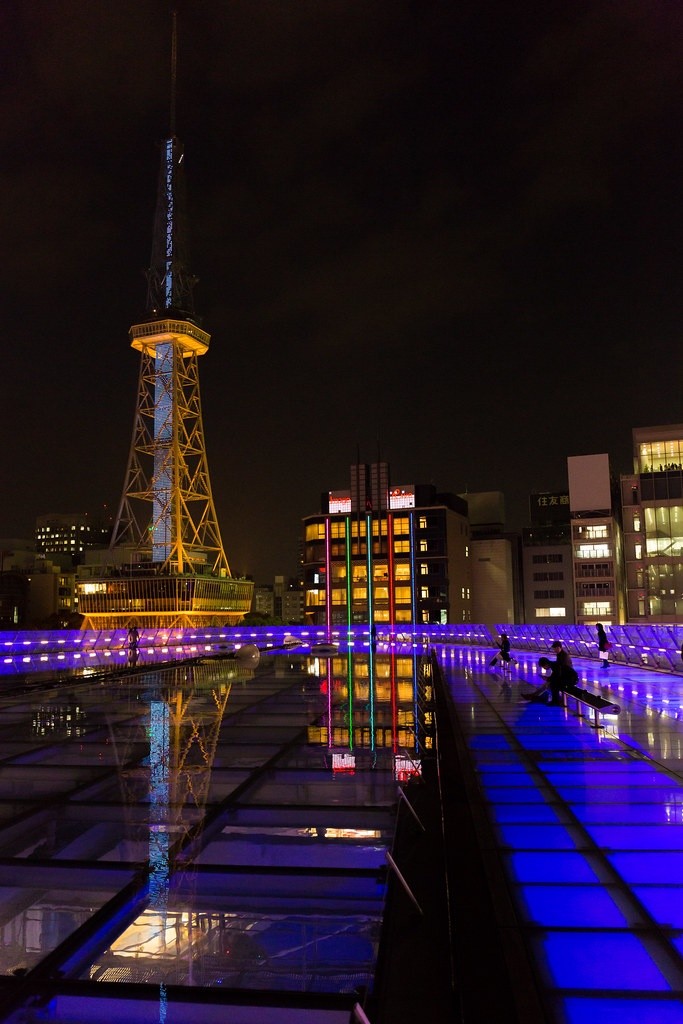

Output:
xmin=488 ymin=633 xmax=510 ymax=666
xmin=127 ymin=626 xmax=140 ymax=649
xmin=659 ymin=463 xmax=682 ymax=471
xmin=595 ymin=623 xmax=612 ymax=669
xmin=521 ymin=641 xmax=579 ymax=706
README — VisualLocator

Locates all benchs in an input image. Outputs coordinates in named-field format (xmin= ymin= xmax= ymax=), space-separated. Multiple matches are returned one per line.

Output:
xmin=560 ymin=685 xmax=621 ymax=729
xmin=501 ymin=657 xmax=513 ymax=668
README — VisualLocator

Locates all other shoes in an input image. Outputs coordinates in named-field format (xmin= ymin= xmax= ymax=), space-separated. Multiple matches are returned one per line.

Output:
xmin=548 ymin=700 xmax=562 ymax=706
xmin=521 ymin=693 xmax=536 ymax=700
xmin=600 ymin=664 xmax=610 ymax=668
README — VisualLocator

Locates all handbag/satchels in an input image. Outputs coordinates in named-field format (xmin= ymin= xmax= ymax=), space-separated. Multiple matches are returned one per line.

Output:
xmin=599 ymin=650 xmax=609 ymax=660
